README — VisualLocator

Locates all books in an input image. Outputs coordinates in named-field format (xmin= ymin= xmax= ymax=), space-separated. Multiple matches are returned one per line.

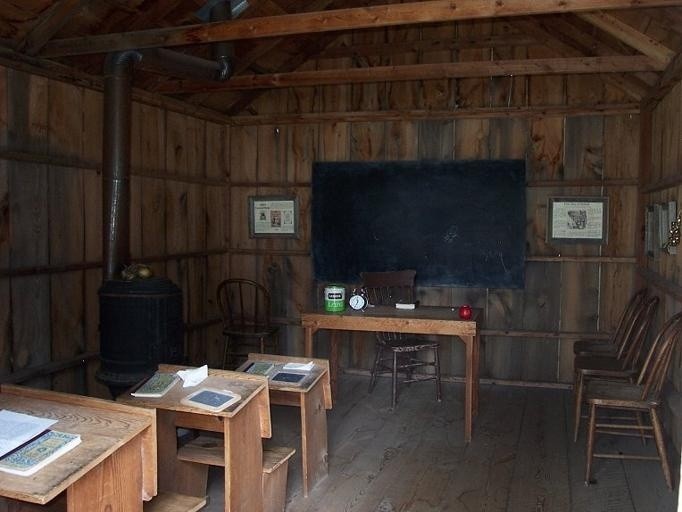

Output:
xmin=243 ymin=362 xmax=274 ymax=375
xmin=132 ymin=371 xmax=180 ymax=397
xmin=395 ymin=298 xmax=419 ymax=310
xmin=1 ymin=430 xmax=82 ymax=474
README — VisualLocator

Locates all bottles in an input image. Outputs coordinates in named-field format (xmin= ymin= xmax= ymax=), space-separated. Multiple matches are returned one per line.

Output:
xmin=459 ymin=305 xmax=472 ymax=320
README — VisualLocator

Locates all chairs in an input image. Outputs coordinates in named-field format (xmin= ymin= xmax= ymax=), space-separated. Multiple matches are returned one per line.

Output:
xmin=573 ymin=295 xmax=660 ymax=446
xmin=361 ymin=270 xmax=442 ymax=408
xmin=584 ymin=310 xmax=682 ymax=494
xmin=573 ymin=288 xmax=648 ymax=403
xmin=216 ymin=278 xmax=280 ymax=371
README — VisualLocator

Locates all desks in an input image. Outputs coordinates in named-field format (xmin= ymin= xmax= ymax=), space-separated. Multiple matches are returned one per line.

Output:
xmin=302 ymin=305 xmax=484 ymax=443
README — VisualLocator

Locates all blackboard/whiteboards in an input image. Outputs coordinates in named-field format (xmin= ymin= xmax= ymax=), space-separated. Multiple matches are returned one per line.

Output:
xmin=270 ymin=371 xmax=308 ymax=385
xmin=310 ymin=159 xmax=526 ymax=288
xmin=180 ymin=386 xmax=242 ymax=414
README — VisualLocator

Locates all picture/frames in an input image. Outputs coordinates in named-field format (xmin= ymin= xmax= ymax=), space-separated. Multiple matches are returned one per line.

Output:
xmin=657 ymin=201 xmax=676 ymax=255
xmin=644 ymin=202 xmax=660 ymax=261
xmin=544 ymin=195 xmax=609 ymax=247
xmin=247 ymin=194 xmax=298 ymax=240
